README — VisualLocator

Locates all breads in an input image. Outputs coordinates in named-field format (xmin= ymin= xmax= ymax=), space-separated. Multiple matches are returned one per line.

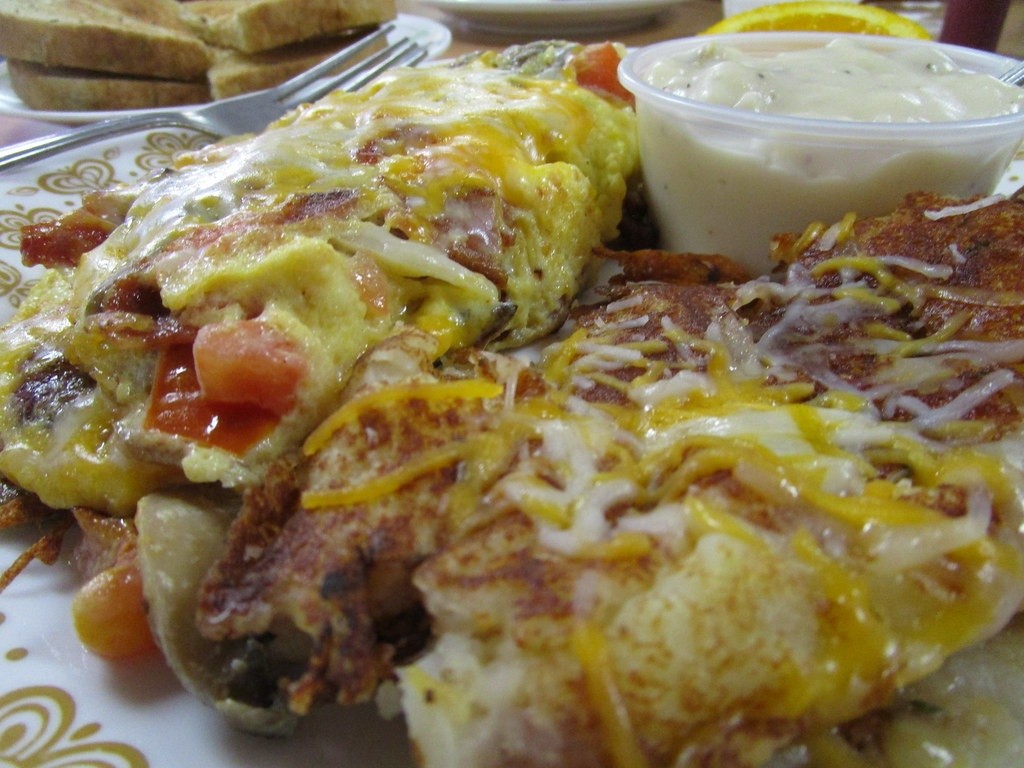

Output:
xmin=0 ymin=0 xmax=402 ymax=108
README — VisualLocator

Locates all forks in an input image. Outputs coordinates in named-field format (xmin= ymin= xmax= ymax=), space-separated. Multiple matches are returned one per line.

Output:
xmin=0 ymin=23 xmax=427 ymax=214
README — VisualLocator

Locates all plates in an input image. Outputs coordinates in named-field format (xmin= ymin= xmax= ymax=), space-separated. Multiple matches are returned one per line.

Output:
xmin=0 ymin=13 xmax=453 ymax=127
xmin=929 ymin=43 xmax=1024 ymax=206
xmin=0 ymin=126 xmax=474 ymax=768
xmin=427 ymin=0 xmax=680 ymax=36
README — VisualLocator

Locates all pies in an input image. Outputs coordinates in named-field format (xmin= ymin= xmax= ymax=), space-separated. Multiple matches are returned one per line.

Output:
xmin=1 ymin=38 xmax=1024 ymax=768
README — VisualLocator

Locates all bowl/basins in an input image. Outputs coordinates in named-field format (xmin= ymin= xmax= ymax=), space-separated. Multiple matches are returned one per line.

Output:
xmin=618 ymin=31 xmax=1023 ymax=273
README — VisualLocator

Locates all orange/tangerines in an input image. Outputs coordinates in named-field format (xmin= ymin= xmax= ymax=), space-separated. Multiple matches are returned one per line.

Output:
xmin=690 ymin=0 xmax=935 ymax=41
